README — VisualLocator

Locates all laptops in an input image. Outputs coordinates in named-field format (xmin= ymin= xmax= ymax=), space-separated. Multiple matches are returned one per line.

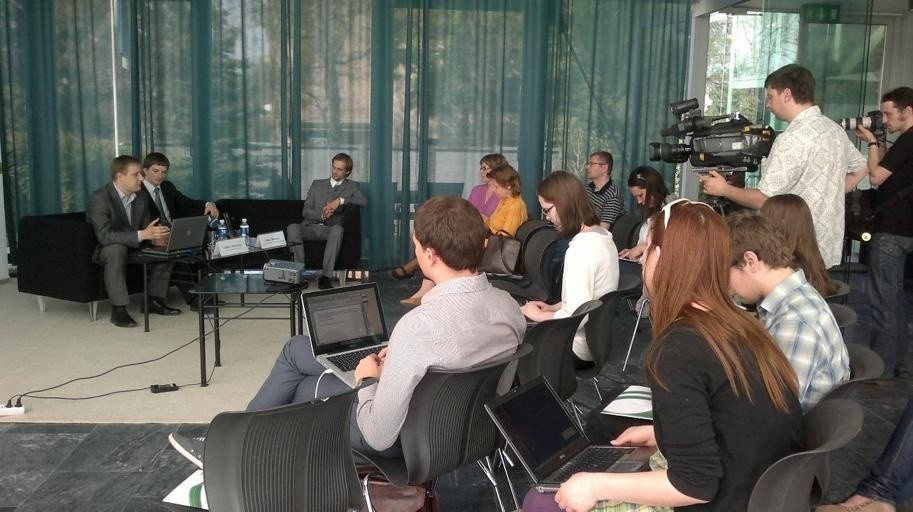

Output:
xmin=300 ymin=281 xmax=390 ymax=390
xmin=141 ymin=216 xmax=208 ymax=256
xmin=483 ymin=374 xmax=658 ymax=494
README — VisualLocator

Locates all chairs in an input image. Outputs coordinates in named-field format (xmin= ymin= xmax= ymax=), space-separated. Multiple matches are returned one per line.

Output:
xmin=575 ymin=258 xmax=642 ymax=403
xmin=826 ymin=302 xmax=857 ymax=337
xmin=612 ymin=214 xmax=644 ymax=253
xmin=516 ymin=300 xmax=602 ymax=414
xmin=820 ymin=343 xmax=884 ymax=400
xmin=351 ymin=343 xmax=533 ymax=512
xmin=487 ymin=229 xmax=561 ymax=302
xmin=822 ymin=279 xmax=851 ymax=301
xmin=746 ymin=399 xmax=863 ymax=512
xmin=143 ymin=378 xmax=377 ymax=512
xmin=485 ymin=219 xmax=554 ymax=287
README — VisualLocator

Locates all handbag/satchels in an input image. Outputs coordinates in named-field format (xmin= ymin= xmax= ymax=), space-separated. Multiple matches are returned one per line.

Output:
xmin=481 ymin=229 xmax=533 ymax=289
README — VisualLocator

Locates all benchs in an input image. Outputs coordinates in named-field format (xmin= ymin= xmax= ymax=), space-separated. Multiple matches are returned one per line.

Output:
xmin=211 ymin=199 xmax=361 ymax=287
xmin=17 ymin=200 xmax=211 ymax=321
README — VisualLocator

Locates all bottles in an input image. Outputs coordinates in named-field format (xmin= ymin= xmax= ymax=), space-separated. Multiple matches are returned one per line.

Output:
xmin=240 ymin=219 xmax=250 ymax=248
xmin=217 ymin=220 xmax=227 ymax=241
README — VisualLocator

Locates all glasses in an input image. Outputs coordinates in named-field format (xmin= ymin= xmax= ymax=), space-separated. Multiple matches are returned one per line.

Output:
xmin=541 ymin=202 xmax=556 ymax=216
xmin=628 ymin=172 xmax=648 ymax=182
xmin=480 ymin=167 xmax=492 ymax=171
xmin=660 ymin=197 xmax=716 ymax=231
xmin=586 ymin=161 xmax=606 ymax=166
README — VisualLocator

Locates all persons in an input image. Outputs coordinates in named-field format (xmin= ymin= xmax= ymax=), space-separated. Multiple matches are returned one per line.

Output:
xmin=288 ymin=152 xmax=367 ymax=290
xmin=392 ymin=154 xmax=528 ymax=305
xmin=854 ymin=87 xmax=912 ymax=386
xmin=815 ymin=393 xmax=913 ymax=512
xmin=86 ymin=155 xmax=181 ymax=328
xmin=140 ymin=152 xmax=225 ymax=314
xmin=522 ymin=198 xmax=806 ymax=512
xmin=724 ymin=194 xmax=851 ymax=413
xmin=697 ymin=64 xmax=869 ymax=270
xmin=167 ymin=196 xmax=527 ymax=471
xmin=519 ymin=151 xmax=676 ymax=371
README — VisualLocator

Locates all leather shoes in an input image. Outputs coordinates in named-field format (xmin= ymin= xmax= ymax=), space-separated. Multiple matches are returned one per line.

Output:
xmin=140 ymin=300 xmax=182 ymax=317
xmin=300 ymin=282 xmax=309 ymax=288
xmin=209 ymin=299 xmax=226 ymax=305
xmin=110 ymin=305 xmax=138 ymax=328
xmin=390 ymin=265 xmax=415 ymax=279
xmin=317 ymin=275 xmax=334 ymax=290
xmin=812 ymin=498 xmax=897 ymax=512
xmin=400 ymin=296 xmax=421 ymax=306
xmin=189 ymin=300 xmax=217 ymax=313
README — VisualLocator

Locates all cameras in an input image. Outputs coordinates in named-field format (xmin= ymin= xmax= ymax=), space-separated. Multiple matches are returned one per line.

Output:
xmin=839 ymin=110 xmax=887 ymax=143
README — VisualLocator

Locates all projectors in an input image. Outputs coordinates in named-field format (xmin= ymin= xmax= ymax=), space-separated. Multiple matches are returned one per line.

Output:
xmin=262 ymin=259 xmax=306 ymax=285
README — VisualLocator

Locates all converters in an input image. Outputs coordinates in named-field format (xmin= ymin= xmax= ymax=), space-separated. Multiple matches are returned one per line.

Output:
xmin=151 ymin=383 xmax=178 ymax=393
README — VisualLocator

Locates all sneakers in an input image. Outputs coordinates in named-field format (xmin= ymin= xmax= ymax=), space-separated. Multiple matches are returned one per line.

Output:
xmin=169 ymin=432 xmax=205 ymax=468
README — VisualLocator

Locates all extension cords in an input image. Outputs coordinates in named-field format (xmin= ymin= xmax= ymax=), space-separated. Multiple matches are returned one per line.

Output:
xmin=0 ymin=403 xmax=25 ymax=416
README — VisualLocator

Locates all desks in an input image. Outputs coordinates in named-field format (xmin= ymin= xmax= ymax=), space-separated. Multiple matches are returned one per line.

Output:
xmin=137 ymin=235 xmax=303 ymax=332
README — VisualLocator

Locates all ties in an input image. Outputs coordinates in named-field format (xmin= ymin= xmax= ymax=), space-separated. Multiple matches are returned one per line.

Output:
xmin=153 ymin=187 xmax=172 ymax=230
